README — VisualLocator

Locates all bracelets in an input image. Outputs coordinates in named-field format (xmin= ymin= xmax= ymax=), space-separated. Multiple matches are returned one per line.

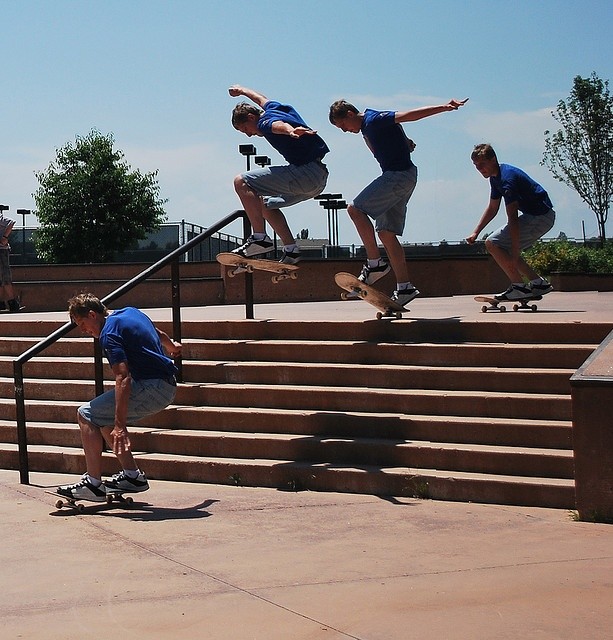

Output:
xmin=2 ymin=235 xmax=8 ymax=239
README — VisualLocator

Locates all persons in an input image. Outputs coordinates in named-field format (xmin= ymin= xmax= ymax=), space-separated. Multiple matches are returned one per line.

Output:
xmin=228 ymin=84 xmax=331 ymax=265
xmin=0 ymin=212 xmax=20 ymax=312
xmin=328 ymin=96 xmax=470 ymax=307
xmin=56 ymin=292 xmax=183 ymax=503
xmin=465 ymin=143 xmax=555 ymax=301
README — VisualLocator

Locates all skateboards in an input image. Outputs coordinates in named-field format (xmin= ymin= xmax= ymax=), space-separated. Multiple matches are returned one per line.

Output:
xmin=335 ymin=273 xmax=410 ymax=318
xmin=45 ymin=490 xmax=135 ymax=511
xmin=217 ymin=252 xmax=300 ymax=283
xmin=475 ymin=294 xmax=544 ymax=314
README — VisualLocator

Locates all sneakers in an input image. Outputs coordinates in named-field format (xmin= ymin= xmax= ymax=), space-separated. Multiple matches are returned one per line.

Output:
xmin=277 ymin=246 xmax=300 ymax=264
xmin=390 ymin=284 xmax=420 ymax=309
xmin=102 ymin=469 xmax=149 ymax=492
xmin=230 ymin=235 xmax=275 ymax=257
xmin=57 ymin=472 xmax=106 ymax=502
xmin=527 ymin=277 xmax=553 ymax=295
xmin=494 ymin=283 xmax=533 ymax=300
xmin=357 ymin=259 xmax=391 ymax=286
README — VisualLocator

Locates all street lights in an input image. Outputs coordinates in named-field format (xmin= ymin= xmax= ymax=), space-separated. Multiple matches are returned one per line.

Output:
xmin=16 ymin=207 xmax=33 ymax=253
xmin=253 ymin=154 xmax=271 ymax=168
xmin=238 ymin=143 xmax=256 ymax=171
xmin=323 ymin=195 xmax=348 ymax=245
xmin=0 ymin=204 xmax=11 ymax=216
xmin=313 ymin=192 xmax=344 ymax=245
xmin=320 ymin=199 xmax=339 ymax=245
